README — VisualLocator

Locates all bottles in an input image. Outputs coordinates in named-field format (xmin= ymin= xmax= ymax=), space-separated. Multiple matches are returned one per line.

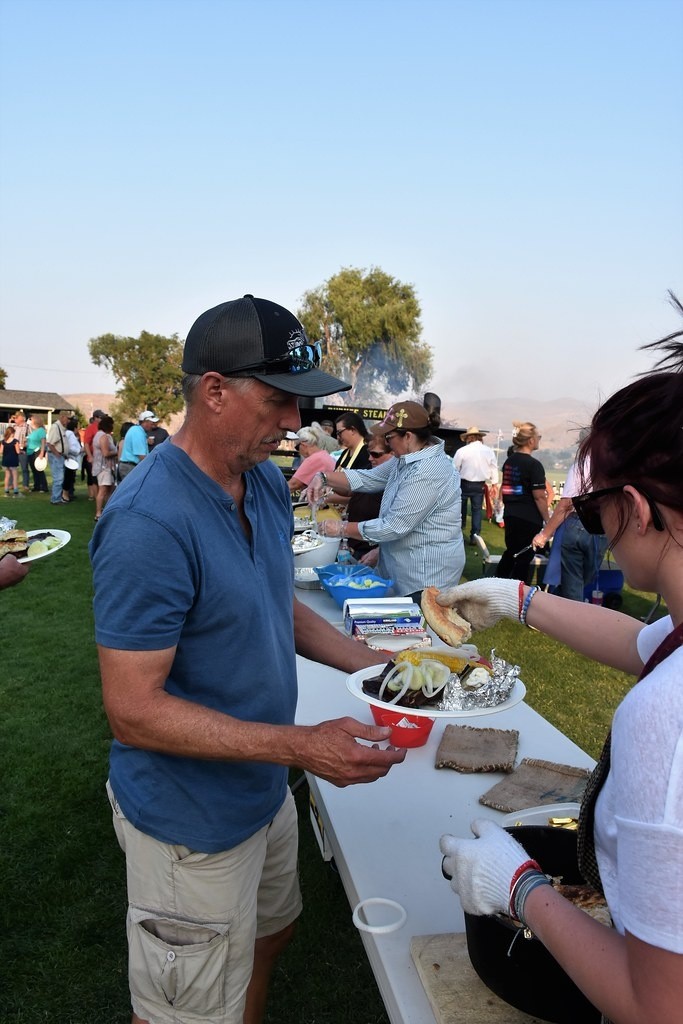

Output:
xmin=558 ymin=482 xmax=564 ymax=495
xmin=552 ymin=481 xmax=557 ymax=494
xmin=337 ymin=538 xmax=351 ymax=564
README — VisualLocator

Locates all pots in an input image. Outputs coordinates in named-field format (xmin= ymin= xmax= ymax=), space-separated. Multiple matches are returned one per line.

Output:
xmin=442 ymin=826 xmax=618 ymax=1024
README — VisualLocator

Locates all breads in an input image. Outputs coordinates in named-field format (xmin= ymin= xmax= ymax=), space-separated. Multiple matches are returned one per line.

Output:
xmin=0 ymin=530 xmax=28 ymax=558
xmin=552 ymin=886 xmax=612 ymax=927
xmin=421 ymin=586 xmax=471 ymax=648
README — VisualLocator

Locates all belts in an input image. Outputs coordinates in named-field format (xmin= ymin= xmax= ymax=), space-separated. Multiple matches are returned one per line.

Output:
xmin=121 ymin=461 xmax=138 ymax=466
xmin=48 ymin=451 xmax=64 ymax=456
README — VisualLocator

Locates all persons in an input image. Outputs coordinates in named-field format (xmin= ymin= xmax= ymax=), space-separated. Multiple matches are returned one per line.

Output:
xmin=496 ymin=420 xmax=556 ymax=586
xmin=287 ymin=412 xmax=394 ymax=569
xmin=532 ymin=428 xmax=609 ymax=603
xmin=452 ymin=426 xmax=498 ymax=546
xmin=0 ymin=427 xmax=25 ymax=498
xmin=46 ymin=411 xmax=86 ymax=505
xmin=116 ymin=410 xmax=170 ymax=485
xmin=8 ymin=411 xmax=49 ymax=493
xmin=306 ymin=401 xmax=466 ymax=610
xmin=0 ymin=554 xmax=31 ymax=590
xmin=89 ymin=294 xmax=407 ymax=1024
xmin=82 ymin=410 xmax=118 ymax=521
xmin=436 ymin=372 xmax=683 ymax=1024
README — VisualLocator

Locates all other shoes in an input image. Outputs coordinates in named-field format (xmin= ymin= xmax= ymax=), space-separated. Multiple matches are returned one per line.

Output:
xmin=50 ymin=499 xmax=67 ymax=504
xmin=61 ymin=495 xmax=71 ymax=503
xmin=23 ymin=485 xmax=30 ymax=491
xmin=88 ymin=497 xmax=97 ymax=500
xmin=94 ymin=515 xmax=100 ymax=521
xmin=39 ymin=490 xmax=44 ymax=494
xmin=469 ymin=535 xmax=478 ymax=545
xmin=13 ymin=492 xmax=26 ymax=498
xmin=3 ymin=491 xmax=13 ymax=497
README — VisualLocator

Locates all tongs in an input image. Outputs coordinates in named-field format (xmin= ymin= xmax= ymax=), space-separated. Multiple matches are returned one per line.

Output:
xmin=513 ymin=543 xmax=533 ymax=558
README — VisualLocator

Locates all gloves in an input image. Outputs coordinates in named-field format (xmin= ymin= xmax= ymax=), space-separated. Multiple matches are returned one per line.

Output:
xmin=440 ymin=818 xmax=542 ymax=920
xmin=437 ymin=575 xmax=525 ymax=632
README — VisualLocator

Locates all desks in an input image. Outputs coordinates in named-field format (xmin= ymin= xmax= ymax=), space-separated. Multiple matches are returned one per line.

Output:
xmin=289 ymin=585 xmax=598 ymax=1024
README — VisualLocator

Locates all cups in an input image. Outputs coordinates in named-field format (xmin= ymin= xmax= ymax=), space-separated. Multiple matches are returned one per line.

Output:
xmin=149 ymin=436 xmax=155 ymax=444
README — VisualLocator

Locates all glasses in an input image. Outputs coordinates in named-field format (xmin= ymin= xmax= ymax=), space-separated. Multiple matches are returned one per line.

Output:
xmin=335 ymin=428 xmax=348 ymax=436
xmin=295 ymin=440 xmax=306 ymax=452
xmin=218 ymin=340 xmax=322 ymax=377
xmin=383 ymin=434 xmax=399 ymax=443
xmin=366 ymin=448 xmax=387 ymax=459
xmin=144 ymin=415 xmax=156 ymax=421
xmin=536 ymin=435 xmax=542 ymax=440
xmin=97 ymin=417 xmax=103 ymax=419
xmin=571 ymin=480 xmax=665 ymax=535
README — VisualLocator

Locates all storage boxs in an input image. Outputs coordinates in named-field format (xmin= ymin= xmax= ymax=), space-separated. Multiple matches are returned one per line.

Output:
xmin=473 ymin=532 xmax=549 ymax=585
xmin=345 ymin=609 xmax=427 ymax=636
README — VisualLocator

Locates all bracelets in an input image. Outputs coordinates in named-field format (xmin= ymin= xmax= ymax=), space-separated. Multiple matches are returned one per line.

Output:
xmin=519 ymin=584 xmax=549 ymax=633
xmin=508 ymin=870 xmax=555 ymax=958
xmin=320 ymin=471 xmax=328 ymax=486
xmin=340 ymin=522 xmax=345 ymax=538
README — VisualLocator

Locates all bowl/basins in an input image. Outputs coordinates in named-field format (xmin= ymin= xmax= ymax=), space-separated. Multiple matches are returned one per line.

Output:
xmin=370 ymin=704 xmax=406 ymax=728
xmin=381 ymin=715 xmax=436 ymax=748
xmin=312 ymin=564 xmax=378 ymax=598
xmin=323 ymin=574 xmax=395 ymax=610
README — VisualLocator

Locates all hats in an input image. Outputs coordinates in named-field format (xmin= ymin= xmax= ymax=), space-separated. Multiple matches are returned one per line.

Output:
xmin=369 ymin=401 xmax=429 ymax=435
xmin=60 ymin=410 xmax=73 ymax=418
xmin=139 ymin=411 xmax=159 ymax=422
xmin=182 ymin=294 xmax=353 ymax=397
xmin=321 ymin=420 xmax=334 ymax=427
xmin=460 ymin=426 xmax=487 ymax=442
xmin=93 ymin=410 xmax=105 ymax=417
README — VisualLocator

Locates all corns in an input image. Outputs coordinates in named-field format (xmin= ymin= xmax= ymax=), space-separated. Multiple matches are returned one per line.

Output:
xmin=395 ymin=650 xmax=493 ymax=675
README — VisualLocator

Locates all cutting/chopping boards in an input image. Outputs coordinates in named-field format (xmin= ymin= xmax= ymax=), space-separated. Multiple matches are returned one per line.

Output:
xmin=410 ymin=932 xmax=555 ymax=1024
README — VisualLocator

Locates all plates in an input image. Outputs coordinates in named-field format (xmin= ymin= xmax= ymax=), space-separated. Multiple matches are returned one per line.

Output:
xmin=34 ymin=457 xmax=47 ymax=472
xmin=17 ymin=529 xmax=71 ymax=564
xmin=346 ymin=663 xmax=527 ymax=717
xmin=64 ymin=459 xmax=79 ymax=470
